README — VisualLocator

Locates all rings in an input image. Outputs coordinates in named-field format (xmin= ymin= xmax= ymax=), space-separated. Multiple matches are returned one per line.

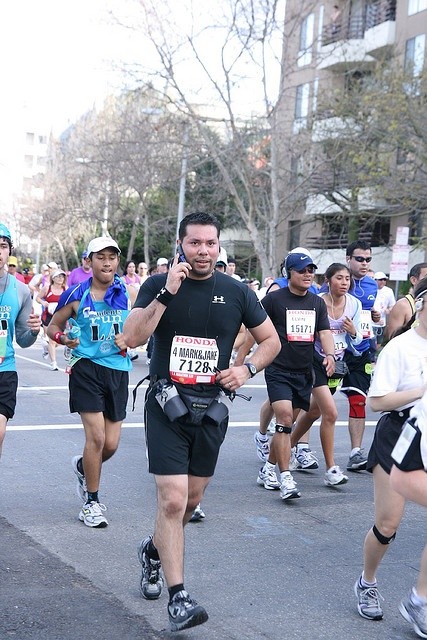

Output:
xmin=228 ymin=383 xmax=231 ymax=387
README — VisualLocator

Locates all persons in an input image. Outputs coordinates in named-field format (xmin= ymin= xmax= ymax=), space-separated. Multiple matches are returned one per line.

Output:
xmin=371 ymin=271 xmax=396 ymax=351
xmin=225 ymin=254 xmax=241 ymax=282
xmin=319 ymin=240 xmax=380 ymax=471
xmin=7 ymin=256 xmax=25 ymax=284
xmin=147 ymin=265 xmax=159 ymax=277
xmin=20 ymin=268 xmax=32 ymax=284
xmin=66 ymin=249 xmax=93 ymax=288
xmin=243 ymin=275 xmax=274 ymax=303
xmin=119 ymin=260 xmax=141 ymax=362
xmin=35 ymin=269 xmax=68 ymax=371
xmin=136 ymin=262 xmax=151 ymax=285
xmin=155 ymin=258 xmax=168 ymax=274
xmin=381 ymin=263 xmax=427 ymax=344
xmin=253 ymin=247 xmax=319 ymax=470
xmin=215 ymin=247 xmax=227 ymax=274
xmin=34 ymin=261 xmax=61 ymax=340
xmin=27 ymin=264 xmax=48 ymax=315
xmin=287 ymin=263 xmax=363 ymax=487
xmin=231 ymin=253 xmax=338 ymax=501
xmin=15 ymin=269 xmax=25 ymax=283
xmin=45 ymin=236 xmax=134 ymax=529
xmin=367 ymin=268 xmax=373 ymax=279
xmin=122 ymin=212 xmax=282 ymax=632
xmin=353 ymin=277 xmax=427 ymax=640
xmin=0 ymin=222 xmax=41 ymax=460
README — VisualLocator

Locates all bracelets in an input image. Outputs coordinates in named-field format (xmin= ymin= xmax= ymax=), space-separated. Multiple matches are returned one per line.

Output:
xmin=326 ymin=354 xmax=336 ymax=363
xmin=349 ymin=331 xmax=356 ymax=339
xmin=52 ymin=331 xmax=65 ymax=345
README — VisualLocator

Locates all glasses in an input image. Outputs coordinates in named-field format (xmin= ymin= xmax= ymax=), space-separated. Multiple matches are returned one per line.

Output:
xmin=351 ymin=256 xmax=372 ymax=263
xmin=376 ymin=278 xmax=386 ymax=281
xmin=138 ymin=267 xmax=147 ymax=270
xmin=291 ymin=268 xmax=315 ymax=273
xmin=9 ymin=265 xmax=16 ymax=267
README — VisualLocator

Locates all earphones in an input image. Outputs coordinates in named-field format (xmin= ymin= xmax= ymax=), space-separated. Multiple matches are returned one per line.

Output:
xmin=346 ymin=259 xmax=350 ymax=263
xmin=326 ymin=282 xmax=329 ymax=287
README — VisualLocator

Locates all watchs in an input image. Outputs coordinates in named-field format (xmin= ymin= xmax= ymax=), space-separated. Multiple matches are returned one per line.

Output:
xmin=243 ymin=363 xmax=256 ymax=378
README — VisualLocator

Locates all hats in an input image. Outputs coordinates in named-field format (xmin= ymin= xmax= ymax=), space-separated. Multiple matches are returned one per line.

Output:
xmin=216 ymin=261 xmax=226 ymax=267
xmin=373 ymin=272 xmax=388 ymax=280
xmin=87 ymin=237 xmax=121 ymax=258
xmin=82 ymin=252 xmax=87 ymax=258
xmin=7 ymin=257 xmax=17 ymax=265
xmin=285 ymin=253 xmax=317 ymax=272
xmin=289 ymin=247 xmax=312 ymax=259
xmin=156 ymin=258 xmax=167 ymax=265
xmin=52 ymin=270 xmax=66 ymax=278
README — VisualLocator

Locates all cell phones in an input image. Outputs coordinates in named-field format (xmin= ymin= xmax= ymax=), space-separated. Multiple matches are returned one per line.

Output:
xmin=176 ymin=245 xmax=186 ymax=262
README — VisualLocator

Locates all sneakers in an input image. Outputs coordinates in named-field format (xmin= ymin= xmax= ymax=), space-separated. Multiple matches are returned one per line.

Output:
xmin=50 ymin=362 xmax=58 ymax=370
xmin=256 ymin=466 xmax=278 ymax=490
xmin=288 ymin=448 xmax=296 ymax=471
xmin=254 ymin=433 xmax=269 ymax=462
xmin=137 ymin=535 xmax=163 ymax=600
xmin=78 ymin=501 xmax=108 ymax=528
xmin=323 ymin=466 xmax=348 ymax=487
xmin=167 ymin=590 xmax=208 ymax=633
xmin=267 ymin=418 xmax=277 ymax=435
xmin=397 ymin=590 xmax=426 ymax=638
xmin=64 ymin=347 xmax=70 ymax=361
xmin=352 ymin=573 xmax=383 ymax=620
xmin=71 ymin=455 xmax=88 ymax=502
xmin=42 ymin=345 xmax=49 ymax=360
xmin=127 ymin=350 xmax=138 ymax=361
xmin=345 ymin=449 xmax=368 ymax=472
xmin=294 ymin=448 xmax=318 ymax=470
xmin=279 ymin=475 xmax=300 ymax=500
xmin=189 ymin=503 xmax=205 ymax=521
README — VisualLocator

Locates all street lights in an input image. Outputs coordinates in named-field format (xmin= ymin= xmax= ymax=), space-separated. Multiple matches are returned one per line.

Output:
xmin=142 ymin=108 xmax=185 ymax=255
xmin=75 ymin=158 xmax=107 ymax=238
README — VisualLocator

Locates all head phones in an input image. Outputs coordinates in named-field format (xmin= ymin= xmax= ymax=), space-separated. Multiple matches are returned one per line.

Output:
xmin=279 ymin=255 xmax=290 ymax=279
xmin=412 ymin=291 xmax=425 ymax=312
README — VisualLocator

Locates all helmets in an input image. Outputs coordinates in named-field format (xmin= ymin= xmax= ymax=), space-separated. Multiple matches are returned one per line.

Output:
xmin=0 ymin=223 xmax=11 ymax=256
